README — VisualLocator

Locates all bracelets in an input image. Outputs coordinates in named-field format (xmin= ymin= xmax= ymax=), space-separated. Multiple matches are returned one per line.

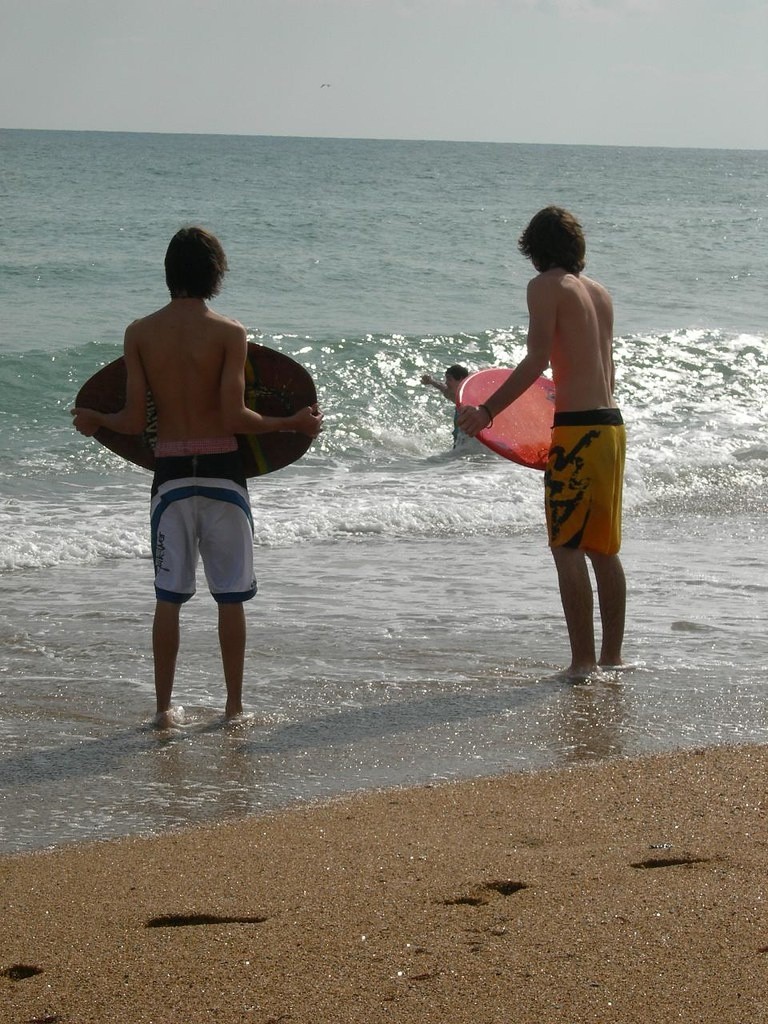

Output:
xmin=477 ymin=404 xmax=493 ymax=429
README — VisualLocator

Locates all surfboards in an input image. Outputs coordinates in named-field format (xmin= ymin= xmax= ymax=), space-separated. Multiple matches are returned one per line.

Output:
xmin=456 ymin=366 xmax=556 ymax=470
xmin=73 ymin=339 xmax=320 ymax=482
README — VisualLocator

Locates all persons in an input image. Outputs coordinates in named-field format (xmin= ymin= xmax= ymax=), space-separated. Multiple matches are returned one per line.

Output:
xmin=421 ymin=364 xmax=469 ymax=447
xmin=72 ymin=228 xmax=326 ymax=731
xmin=455 ymin=206 xmax=628 ymax=676
